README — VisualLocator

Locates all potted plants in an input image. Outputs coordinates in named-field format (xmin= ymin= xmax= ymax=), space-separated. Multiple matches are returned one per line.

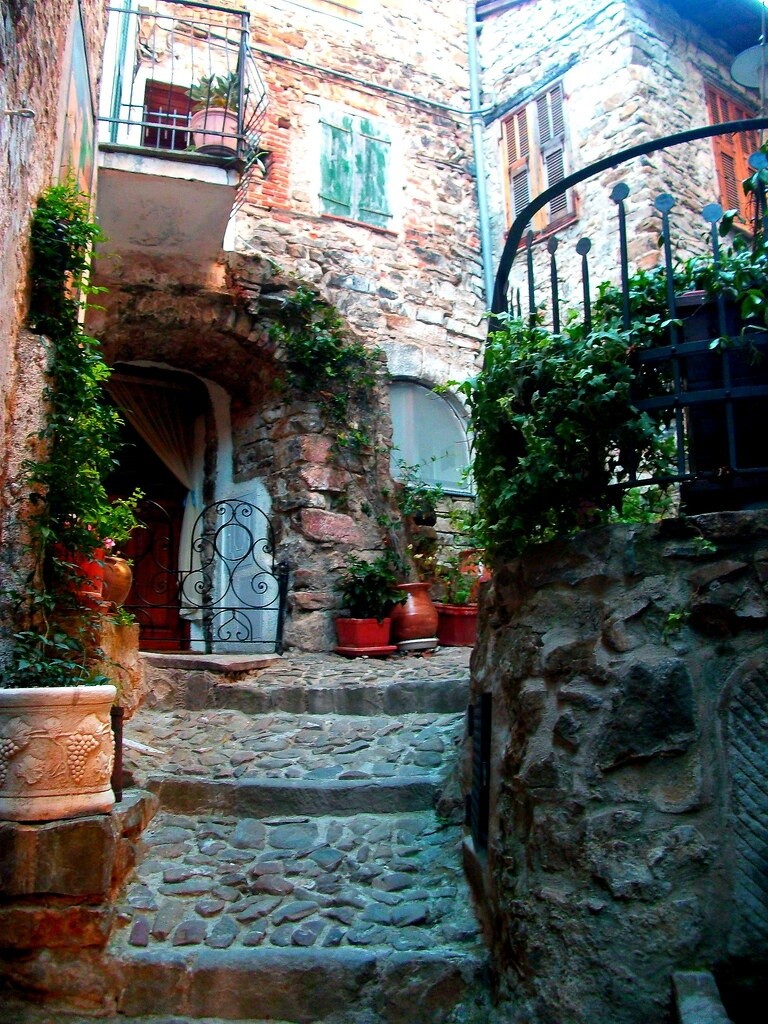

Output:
xmin=183 ymin=70 xmax=251 ymax=157
xmin=334 ymin=552 xmax=409 ymax=657
xmin=0 ymin=619 xmax=116 ymax=823
xmin=103 ymin=486 xmax=148 ymax=617
xmin=432 ymin=555 xmax=479 ymax=648
xmin=454 ymin=506 xmax=494 ymax=602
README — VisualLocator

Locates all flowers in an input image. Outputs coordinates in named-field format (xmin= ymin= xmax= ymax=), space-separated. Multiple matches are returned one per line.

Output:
xmin=26 ymin=510 xmax=120 ymax=566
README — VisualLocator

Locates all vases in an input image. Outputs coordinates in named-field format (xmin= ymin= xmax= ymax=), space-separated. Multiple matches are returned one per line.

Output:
xmin=390 ymin=581 xmax=439 ymax=642
xmin=55 ymin=543 xmax=106 ymax=616
